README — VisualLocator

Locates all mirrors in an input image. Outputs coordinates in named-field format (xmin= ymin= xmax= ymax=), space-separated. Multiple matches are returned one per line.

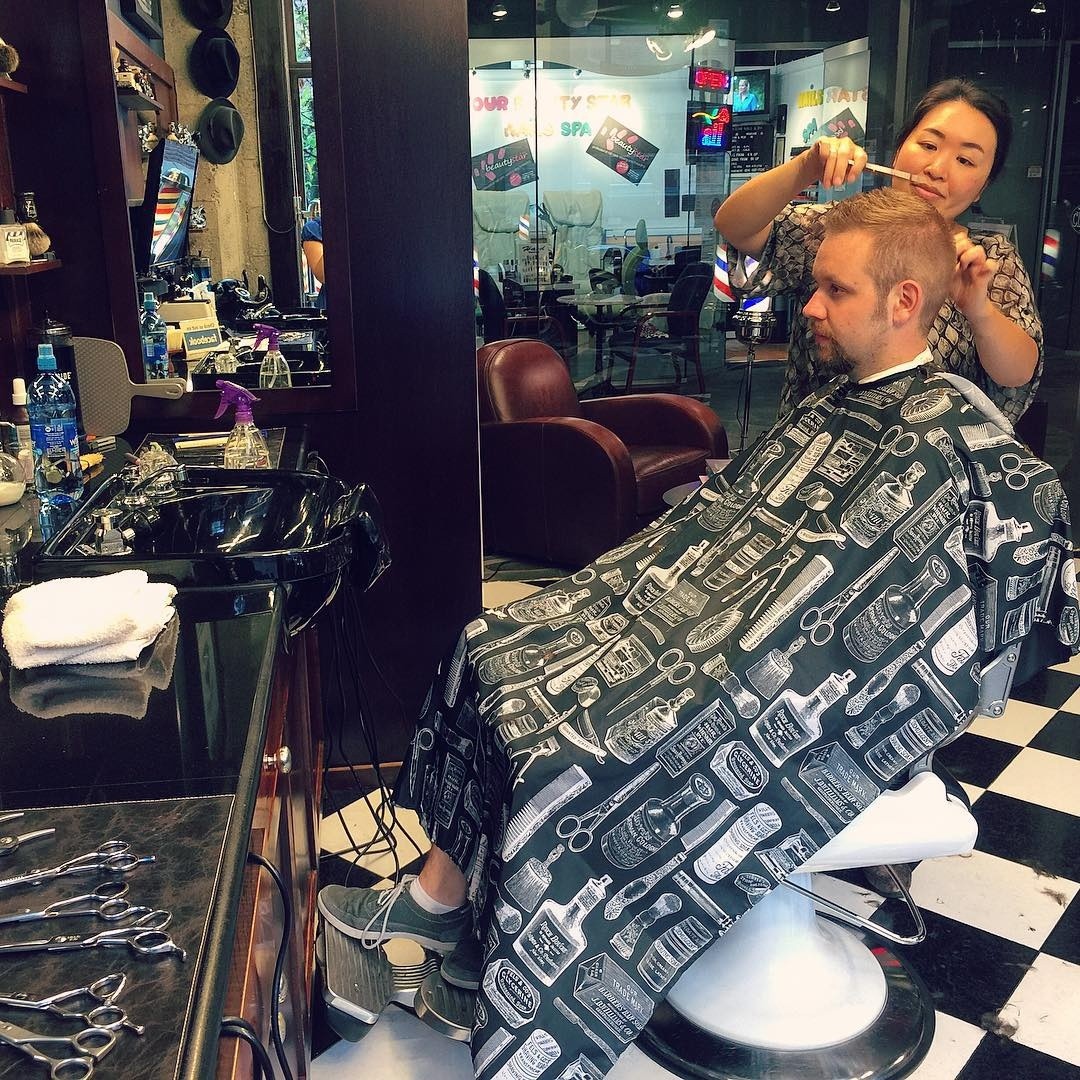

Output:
xmin=140 ymin=1 xmax=356 ymax=410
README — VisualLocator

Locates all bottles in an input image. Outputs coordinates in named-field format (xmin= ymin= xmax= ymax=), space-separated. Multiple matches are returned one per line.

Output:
xmin=25 ymin=344 xmax=84 ymax=504
xmin=1 ymin=209 xmax=31 ymax=264
xmin=141 ymin=293 xmax=169 ymax=381
xmin=6 ymin=377 xmax=34 ymax=483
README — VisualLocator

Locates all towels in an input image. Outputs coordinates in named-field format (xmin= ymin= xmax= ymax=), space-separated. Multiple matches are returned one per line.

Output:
xmin=8 ymin=614 xmax=180 ymax=719
xmin=1 ymin=569 xmax=178 ymax=670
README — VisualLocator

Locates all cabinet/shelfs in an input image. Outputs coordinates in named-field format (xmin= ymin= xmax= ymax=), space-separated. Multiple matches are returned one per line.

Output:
xmin=0 ymin=0 xmax=180 ymax=418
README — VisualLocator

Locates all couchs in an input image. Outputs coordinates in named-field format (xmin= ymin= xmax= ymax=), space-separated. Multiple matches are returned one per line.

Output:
xmin=474 ymin=335 xmax=733 ymax=567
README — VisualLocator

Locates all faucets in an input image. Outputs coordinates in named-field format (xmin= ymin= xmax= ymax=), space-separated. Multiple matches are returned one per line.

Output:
xmin=233 ymin=349 xmax=255 ymax=360
xmin=252 ymin=302 xmax=276 ymax=320
xmin=120 ymin=463 xmax=189 ymax=511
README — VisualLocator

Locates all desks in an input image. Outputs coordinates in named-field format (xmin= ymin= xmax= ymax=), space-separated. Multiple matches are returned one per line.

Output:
xmin=0 ymin=419 xmax=320 ymax=1079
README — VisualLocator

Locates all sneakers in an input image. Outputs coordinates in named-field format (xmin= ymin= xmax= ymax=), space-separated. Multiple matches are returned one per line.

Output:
xmin=317 ymin=873 xmax=473 ymax=952
xmin=440 ymin=932 xmax=484 ymax=990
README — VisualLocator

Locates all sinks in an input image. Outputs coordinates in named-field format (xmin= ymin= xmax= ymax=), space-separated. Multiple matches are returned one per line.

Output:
xmin=233 ymin=302 xmax=328 ymax=330
xmin=31 ymin=468 xmax=353 ymax=637
xmin=191 ymin=350 xmax=332 ymax=391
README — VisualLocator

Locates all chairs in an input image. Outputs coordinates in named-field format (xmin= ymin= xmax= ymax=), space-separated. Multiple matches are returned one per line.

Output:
xmin=478 ymin=239 xmax=714 ymax=400
xmin=634 ymin=753 xmax=978 ymax=1080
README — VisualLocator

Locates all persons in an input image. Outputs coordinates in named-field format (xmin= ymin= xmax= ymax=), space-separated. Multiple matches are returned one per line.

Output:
xmin=733 ymin=78 xmax=759 ymax=113
xmin=300 ymin=213 xmax=327 ymax=310
xmin=713 ymin=76 xmax=1048 ymax=433
xmin=317 ymin=184 xmax=967 ymax=990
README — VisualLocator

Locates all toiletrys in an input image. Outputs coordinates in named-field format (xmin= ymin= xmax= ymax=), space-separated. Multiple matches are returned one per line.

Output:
xmin=250 ymin=323 xmax=293 ymax=388
xmin=213 ymin=379 xmax=271 ymax=469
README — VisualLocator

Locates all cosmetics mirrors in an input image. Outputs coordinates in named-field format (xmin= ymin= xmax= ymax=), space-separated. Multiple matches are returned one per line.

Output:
xmin=70 ymin=335 xmax=184 ymax=437
xmin=146 ymin=377 xmax=188 ymax=393
